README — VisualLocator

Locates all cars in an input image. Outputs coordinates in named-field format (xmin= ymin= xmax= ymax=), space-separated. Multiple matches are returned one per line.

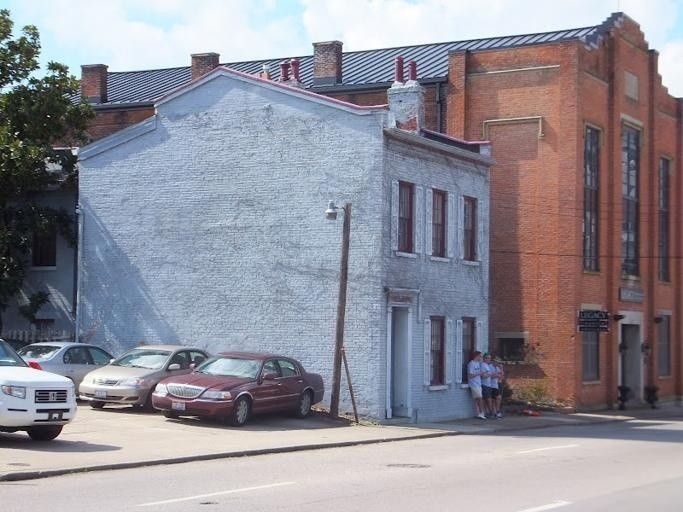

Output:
xmin=76 ymin=342 xmax=216 ymax=413
xmin=16 ymin=342 xmax=116 ymax=399
xmin=151 ymin=352 xmax=325 ymax=426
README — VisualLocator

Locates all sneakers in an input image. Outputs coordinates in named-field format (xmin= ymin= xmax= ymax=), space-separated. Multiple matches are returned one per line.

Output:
xmin=478 ymin=411 xmax=502 ymax=419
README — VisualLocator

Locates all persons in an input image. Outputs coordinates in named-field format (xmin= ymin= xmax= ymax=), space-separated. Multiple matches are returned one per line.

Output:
xmin=490 ymin=356 xmax=504 ymax=418
xmin=465 ymin=350 xmax=489 ymax=419
xmin=481 ymin=351 xmax=500 ymax=419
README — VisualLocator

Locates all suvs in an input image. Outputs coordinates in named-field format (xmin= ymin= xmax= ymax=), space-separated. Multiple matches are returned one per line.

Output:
xmin=0 ymin=336 xmax=76 ymax=444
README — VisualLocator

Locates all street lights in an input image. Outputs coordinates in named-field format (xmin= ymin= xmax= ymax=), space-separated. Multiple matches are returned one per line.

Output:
xmin=324 ymin=199 xmax=353 ymax=419
xmin=641 ymin=340 xmax=658 ymax=408
xmin=617 ymin=341 xmax=629 ymax=410
